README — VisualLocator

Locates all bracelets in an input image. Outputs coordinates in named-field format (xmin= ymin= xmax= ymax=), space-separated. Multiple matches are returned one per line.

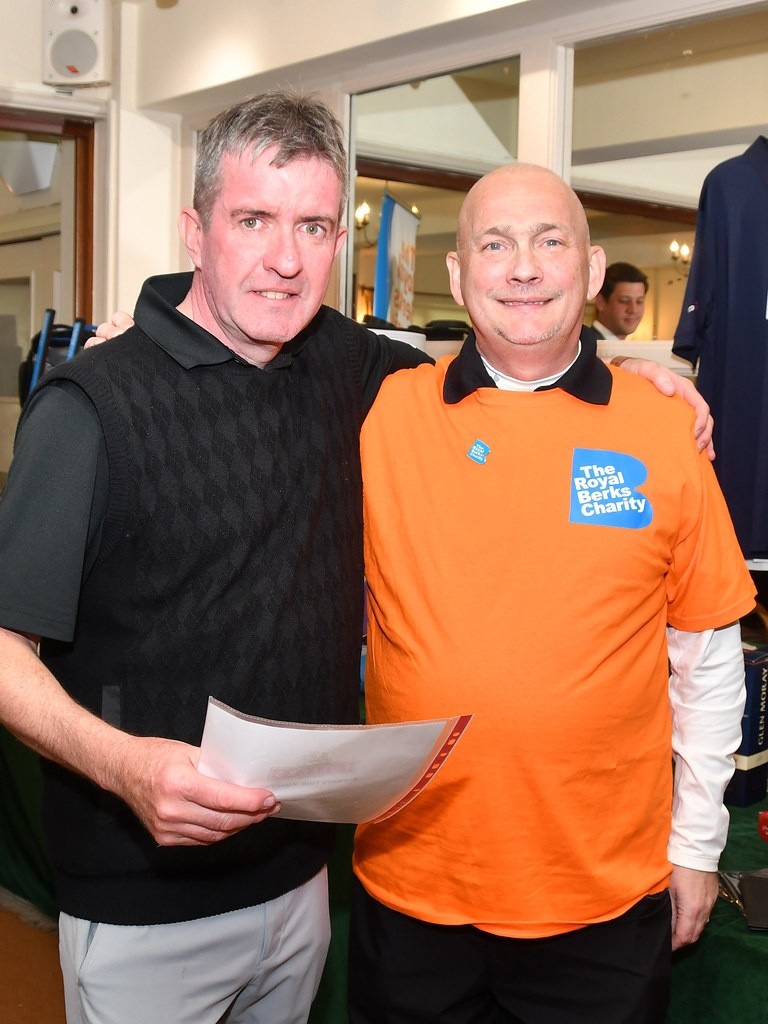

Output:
xmin=609 ymin=355 xmax=631 ymax=367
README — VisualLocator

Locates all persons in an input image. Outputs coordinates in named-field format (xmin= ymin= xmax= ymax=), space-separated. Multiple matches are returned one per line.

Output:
xmin=0 ymin=86 xmax=716 ymax=1024
xmin=82 ymin=163 xmax=757 ymax=1024
xmin=590 ymin=261 xmax=649 ymax=342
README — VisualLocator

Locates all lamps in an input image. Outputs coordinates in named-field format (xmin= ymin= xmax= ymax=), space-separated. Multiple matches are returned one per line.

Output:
xmin=356 ymin=180 xmax=420 ymax=246
xmin=670 ymin=240 xmax=691 ymax=278
